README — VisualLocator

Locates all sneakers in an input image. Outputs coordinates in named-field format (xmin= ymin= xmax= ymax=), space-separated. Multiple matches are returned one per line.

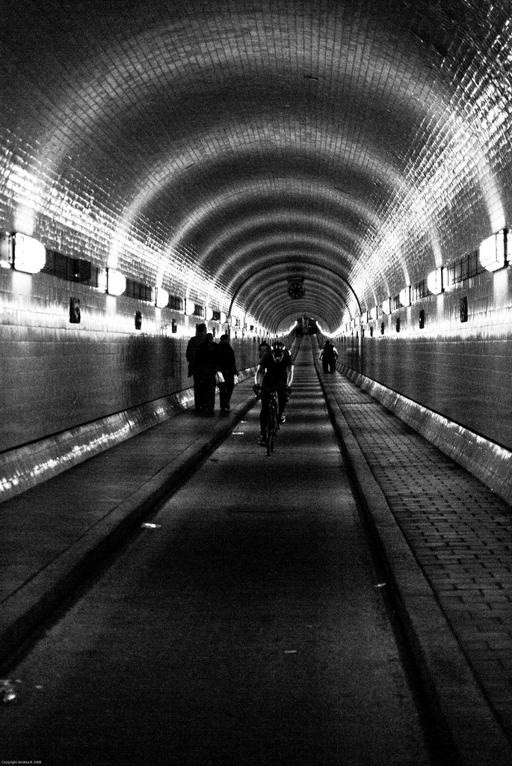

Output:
xmin=257 ymin=435 xmax=267 ymax=444
xmin=279 ymin=411 xmax=286 ymax=424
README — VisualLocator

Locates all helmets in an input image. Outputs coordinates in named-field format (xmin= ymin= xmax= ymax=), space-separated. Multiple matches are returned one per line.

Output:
xmin=270 ymin=340 xmax=285 ymax=362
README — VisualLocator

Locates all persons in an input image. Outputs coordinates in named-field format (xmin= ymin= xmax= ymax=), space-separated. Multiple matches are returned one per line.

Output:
xmin=252 ymin=341 xmax=296 ymax=424
xmin=258 ymin=341 xmax=270 ymax=358
xmin=319 ymin=339 xmax=338 ymax=374
xmin=185 ymin=323 xmax=239 ymax=417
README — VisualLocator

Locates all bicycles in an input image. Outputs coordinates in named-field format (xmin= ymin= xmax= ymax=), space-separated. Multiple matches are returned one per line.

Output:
xmin=256 ymin=389 xmax=291 ymax=457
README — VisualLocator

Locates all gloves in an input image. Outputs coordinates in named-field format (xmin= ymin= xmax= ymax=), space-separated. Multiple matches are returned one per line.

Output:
xmin=285 ymin=386 xmax=291 ymax=394
xmin=253 ymin=383 xmax=260 ymax=394
